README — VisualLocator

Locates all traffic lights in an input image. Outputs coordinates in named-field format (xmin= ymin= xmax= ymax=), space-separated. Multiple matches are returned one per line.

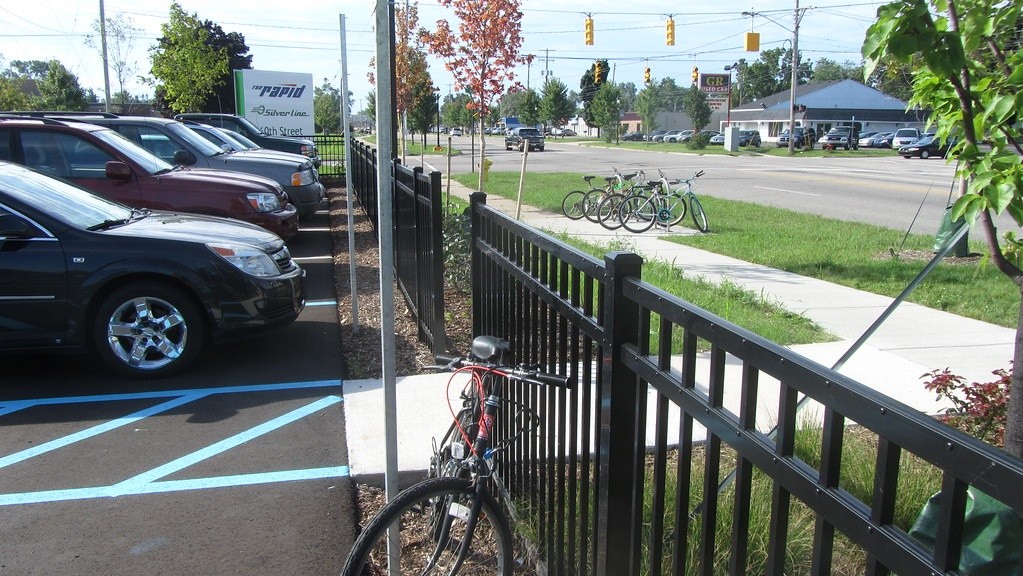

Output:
xmin=693 ymin=66 xmax=699 ymax=82
xmin=644 ymin=67 xmax=651 ymax=83
xmin=595 ymin=61 xmax=601 ymax=84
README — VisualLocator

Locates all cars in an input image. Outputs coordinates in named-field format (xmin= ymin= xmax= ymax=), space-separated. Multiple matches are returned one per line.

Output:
xmin=0 ymin=161 xmax=308 ymax=379
xmin=898 ymin=136 xmax=963 ymax=159
xmin=621 ymin=130 xmax=724 ymax=145
xmin=476 ymin=127 xmax=513 ymax=135
xmin=859 ymin=131 xmax=896 ymax=148
xmin=557 ymin=129 xmax=577 ymax=136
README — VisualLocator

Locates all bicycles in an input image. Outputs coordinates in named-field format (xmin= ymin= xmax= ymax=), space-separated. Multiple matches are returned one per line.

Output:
xmin=562 ymin=167 xmax=687 ymax=230
xmin=340 ymin=336 xmax=576 ymax=576
xmin=618 ymin=170 xmax=708 ymax=234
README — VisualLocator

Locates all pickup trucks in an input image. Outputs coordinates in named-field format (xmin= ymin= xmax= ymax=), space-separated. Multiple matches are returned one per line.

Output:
xmin=504 ymin=127 xmax=545 ymax=151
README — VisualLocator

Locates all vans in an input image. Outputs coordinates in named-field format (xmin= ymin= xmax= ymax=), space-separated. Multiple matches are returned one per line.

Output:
xmin=892 ymin=128 xmax=922 ymax=150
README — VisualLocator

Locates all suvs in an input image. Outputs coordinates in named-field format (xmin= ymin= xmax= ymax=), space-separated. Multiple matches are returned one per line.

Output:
xmin=776 ymin=126 xmax=816 ymax=149
xmin=449 ymin=128 xmax=462 ymax=137
xmin=739 ymin=130 xmax=761 ymax=147
xmin=818 ymin=126 xmax=859 ymax=150
xmin=0 ymin=112 xmax=325 ymax=236
